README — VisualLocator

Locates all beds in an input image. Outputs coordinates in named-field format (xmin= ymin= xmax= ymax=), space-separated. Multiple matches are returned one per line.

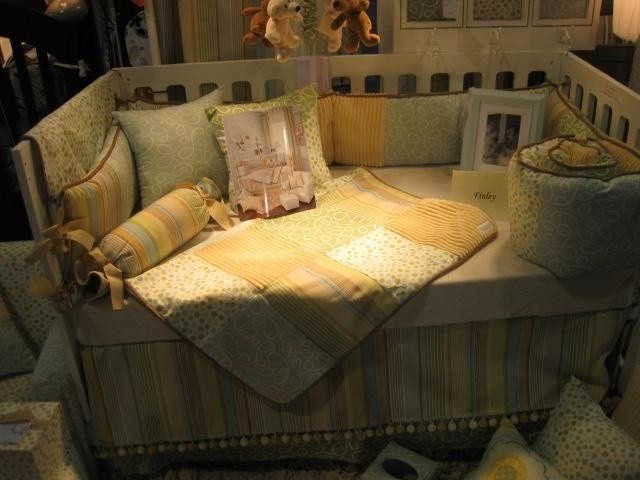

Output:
xmin=10 ymin=45 xmax=640 ymax=480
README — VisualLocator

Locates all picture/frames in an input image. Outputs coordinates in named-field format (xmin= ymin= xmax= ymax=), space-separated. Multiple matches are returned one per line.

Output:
xmin=459 ymin=83 xmax=548 ymax=173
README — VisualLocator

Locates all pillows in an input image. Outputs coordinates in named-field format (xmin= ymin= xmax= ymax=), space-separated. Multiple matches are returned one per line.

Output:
xmin=25 ymin=105 xmax=137 ymax=298
xmin=73 ymin=176 xmax=241 ymax=311
xmin=110 ymin=85 xmax=229 ymax=209
xmin=477 ymin=367 xmax=640 ymax=480
xmin=203 ymin=81 xmax=337 ymax=217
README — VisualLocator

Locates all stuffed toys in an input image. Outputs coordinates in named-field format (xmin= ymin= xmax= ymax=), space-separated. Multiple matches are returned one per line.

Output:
xmin=241 ymin=0 xmax=275 ymax=49
xmin=303 ymin=0 xmax=328 ymax=55
xmin=331 ymin=0 xmax=381 ymax=53
xmin=316 ymin=0 xmax=346 ymax=52
xmin=264 ymin=0 xmax=304 ymax=63
